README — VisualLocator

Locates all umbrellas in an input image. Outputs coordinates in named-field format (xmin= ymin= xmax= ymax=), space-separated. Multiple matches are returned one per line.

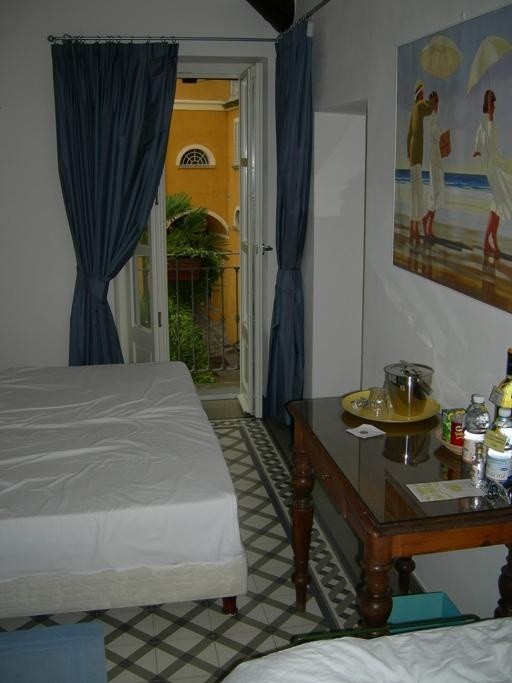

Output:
xmin=418 ymin=32 xmax=462 ymax=80
xmin=465 ymin=33 xmax=511 ymax=94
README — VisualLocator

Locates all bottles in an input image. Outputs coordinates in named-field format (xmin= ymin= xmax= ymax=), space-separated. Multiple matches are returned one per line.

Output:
xmin=463 ymin=349 xmax=512 ymax=490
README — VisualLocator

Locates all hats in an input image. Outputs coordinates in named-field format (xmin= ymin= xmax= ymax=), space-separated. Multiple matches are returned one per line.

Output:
xmin=411 ymin=77 xmax=427 ymax=101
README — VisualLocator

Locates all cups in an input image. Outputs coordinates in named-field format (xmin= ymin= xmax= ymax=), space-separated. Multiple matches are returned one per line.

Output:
xmin=367 ymin=389 xmax=390 ymax=419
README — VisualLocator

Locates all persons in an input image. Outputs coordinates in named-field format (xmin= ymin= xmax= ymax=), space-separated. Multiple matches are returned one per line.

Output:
xmin=471 ymin=89 xmax=511 ymax=257
xmin=422 ymin=90 xmax=444 ymax=236
xmin=404 ymin=77 xmax=435 ymax=241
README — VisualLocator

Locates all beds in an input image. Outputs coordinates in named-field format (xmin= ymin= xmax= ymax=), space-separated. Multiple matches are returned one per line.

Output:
xmin=0 ymin=359 xmax=244 ymax=615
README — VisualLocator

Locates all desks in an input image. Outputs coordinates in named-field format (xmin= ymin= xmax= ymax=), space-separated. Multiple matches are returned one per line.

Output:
xmin=287 ymin=395 xmax=511 ymax=629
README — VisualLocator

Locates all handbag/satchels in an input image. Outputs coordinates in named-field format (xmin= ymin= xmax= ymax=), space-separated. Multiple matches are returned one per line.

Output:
xmin=436 ymin=127 xmax=452 ymax=159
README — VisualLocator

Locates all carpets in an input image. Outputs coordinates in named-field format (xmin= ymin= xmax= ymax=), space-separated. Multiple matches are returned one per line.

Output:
xmin=0 ymin=622 xmax=109 ymax=683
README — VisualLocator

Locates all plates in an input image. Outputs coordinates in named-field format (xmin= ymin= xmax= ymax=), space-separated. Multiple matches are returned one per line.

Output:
xmin=343 ymin=388 xmax=441 ymax=424
